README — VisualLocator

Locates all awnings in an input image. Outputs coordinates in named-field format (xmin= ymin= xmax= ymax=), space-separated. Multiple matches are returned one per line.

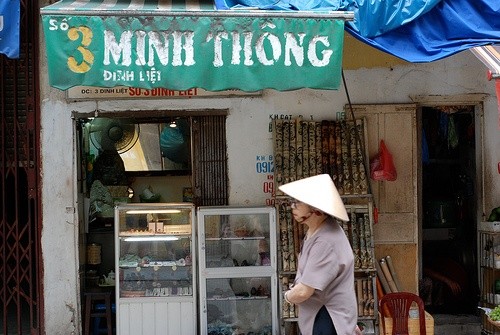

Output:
xmin=40 ymin=0 xmax=354 ymax=93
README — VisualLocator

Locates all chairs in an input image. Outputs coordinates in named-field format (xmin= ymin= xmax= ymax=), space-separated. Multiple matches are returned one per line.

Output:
xmin=380 ymin=292 xmax=426 ymax=335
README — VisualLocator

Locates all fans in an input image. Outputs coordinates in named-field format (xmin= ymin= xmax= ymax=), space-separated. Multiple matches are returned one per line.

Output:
xmin=159 ymin=118 xmax=192 ymax=168
xmin=89 ymin=116 xmax=141 ymax=172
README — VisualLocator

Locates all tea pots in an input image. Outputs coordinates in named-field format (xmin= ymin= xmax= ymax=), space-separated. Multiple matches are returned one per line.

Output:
xmin=103 ymin=270 xmax=115 ymax=284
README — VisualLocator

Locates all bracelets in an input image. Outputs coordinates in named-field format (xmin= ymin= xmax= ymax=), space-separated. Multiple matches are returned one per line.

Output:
xmin=284 ymin=290 xmax=292 ymax=303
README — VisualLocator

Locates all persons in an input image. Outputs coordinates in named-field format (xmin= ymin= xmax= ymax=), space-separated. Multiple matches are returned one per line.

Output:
xmin=423 ymin=257 xmax=462 ymax=314
xmin=277 ymin=174 xmax=358 ymax=335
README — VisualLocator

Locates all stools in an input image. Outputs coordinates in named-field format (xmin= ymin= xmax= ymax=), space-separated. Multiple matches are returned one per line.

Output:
xmin=84 ymin=287 xmax=111 ymax=335
xmin=95 ymin=304 xmax=115 ymax=335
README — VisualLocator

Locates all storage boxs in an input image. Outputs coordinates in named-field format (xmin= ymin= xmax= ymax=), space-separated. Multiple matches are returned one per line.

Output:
xmin=380 ymin=308 xmax=435 ymax=335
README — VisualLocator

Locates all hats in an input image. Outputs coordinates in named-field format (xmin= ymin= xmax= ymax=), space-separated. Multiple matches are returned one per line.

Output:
xmin=278 ymin=174 xmax=349 ymax=222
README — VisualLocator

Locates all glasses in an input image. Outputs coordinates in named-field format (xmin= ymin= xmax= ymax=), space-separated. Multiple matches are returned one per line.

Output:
xmin=290 ymin=200 xmax=304 ymax=207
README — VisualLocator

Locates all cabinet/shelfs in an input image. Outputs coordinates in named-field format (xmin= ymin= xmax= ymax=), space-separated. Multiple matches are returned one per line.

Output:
xmin=278 ymin=194 xmax=378 ymax=335
xmin=115 ymin=202 xmax=197 ymax=334
xmin=198 ymin=206 xmax=277 ymax=335
